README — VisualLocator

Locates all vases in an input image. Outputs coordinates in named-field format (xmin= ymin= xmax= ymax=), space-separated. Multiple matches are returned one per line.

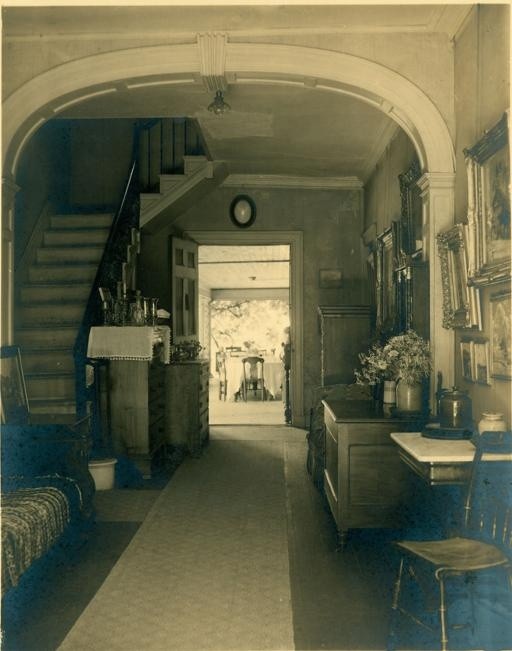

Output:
xmin=380 ymin=378 xmax=424 ymax=413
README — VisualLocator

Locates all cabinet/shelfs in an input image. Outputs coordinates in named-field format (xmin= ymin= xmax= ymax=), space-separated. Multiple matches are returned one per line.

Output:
xmin=110 ymin=326 xmax=210 ymax=479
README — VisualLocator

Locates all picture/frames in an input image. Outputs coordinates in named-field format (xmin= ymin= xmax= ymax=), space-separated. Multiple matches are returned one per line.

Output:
xmin=368 ymin=153 xmax=422 ymax=349
xmin=488 ymin=290 xmax=510 ymax=380
xmin=0 ymin=346 xmax=31 ymax=426
xmin=459 ymin=335 xmax=476 ymax=382
xmin=435 ymin=222 xmax=484 ymax=330
xmin=473 ymin=337 xmax=491 ymax=386
xmin=462 ymin=109 xmax=511 ymax=303
xmin=319 ymin=268 xmax=345 ymax=290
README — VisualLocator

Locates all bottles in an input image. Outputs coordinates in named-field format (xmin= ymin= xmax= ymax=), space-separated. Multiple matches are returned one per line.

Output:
xmin=132 ymin=291 xmax=144 ymax=326
xmin=478 ymin=412 xmax=506 ymax=435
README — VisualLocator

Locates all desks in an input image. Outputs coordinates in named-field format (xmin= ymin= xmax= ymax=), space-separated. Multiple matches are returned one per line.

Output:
xmin=388 ymin=434 xmax=512 ymax=488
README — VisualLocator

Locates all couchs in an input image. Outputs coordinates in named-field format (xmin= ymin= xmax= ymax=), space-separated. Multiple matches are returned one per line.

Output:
xmin=1 ymin=423 xmax=100 ymax=651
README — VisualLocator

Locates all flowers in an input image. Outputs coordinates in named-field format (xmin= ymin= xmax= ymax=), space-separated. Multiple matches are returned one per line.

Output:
xmin=345 ymin=327 xmax=434 ymax=389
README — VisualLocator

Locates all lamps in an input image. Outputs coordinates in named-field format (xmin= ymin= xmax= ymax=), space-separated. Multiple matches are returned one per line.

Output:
xmin=207 ymin=91 xmax=233 ymax=117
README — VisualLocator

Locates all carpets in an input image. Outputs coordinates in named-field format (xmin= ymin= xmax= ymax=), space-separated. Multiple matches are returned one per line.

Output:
xmin=56 ymin=441 xmax=295 ymax=651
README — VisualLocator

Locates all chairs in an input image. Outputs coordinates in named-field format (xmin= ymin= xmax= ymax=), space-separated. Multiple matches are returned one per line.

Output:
xmin=240 ymin=357 xmax=265 ymax=404
xmin=384 ymin=432 xmax=511 ymax=651
